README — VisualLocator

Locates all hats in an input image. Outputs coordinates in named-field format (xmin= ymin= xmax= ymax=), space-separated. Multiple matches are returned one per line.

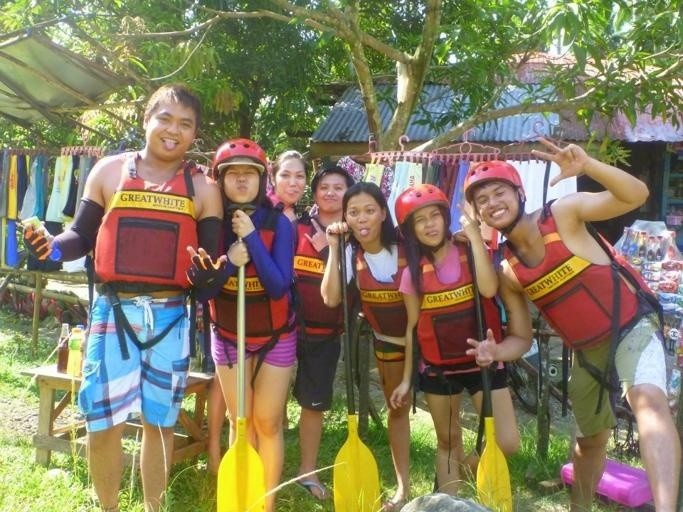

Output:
xmin=217 ymin=158 xmax=266 ymax=176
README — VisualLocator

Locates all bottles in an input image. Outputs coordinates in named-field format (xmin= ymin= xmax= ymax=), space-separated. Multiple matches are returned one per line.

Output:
xmin=56 ymin=321 xmax=85 ymax=379
xmin=621 ymin=227 xmax=676 ymax=262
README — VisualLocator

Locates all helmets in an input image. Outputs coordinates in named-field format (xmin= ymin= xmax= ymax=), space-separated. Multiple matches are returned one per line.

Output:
xmin=463 ymin=160 xmax=524 ymax=205
xmin=212 ymin=138 xmax=268 ymax=170
xmin=395 ymin=184 xmax=451 ymax=225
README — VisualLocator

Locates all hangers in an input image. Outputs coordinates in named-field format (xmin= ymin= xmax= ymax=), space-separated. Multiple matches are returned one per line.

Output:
xmin=184 ymin=138 xmax=214 ymax=175
xmin=350 ymin=121 xmax=571 ymax=158
xmin=61 ymin=135 xmax=105 ymax=158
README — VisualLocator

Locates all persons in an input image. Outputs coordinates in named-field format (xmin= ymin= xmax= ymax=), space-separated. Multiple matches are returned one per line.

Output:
xmin=318 ymin=181 xmax=464 ymax=512
xmin=399 ymin=492 xmax=492 ymax=512
xmin=288 ymin=164 xmax=353 ymax=500
xmin=394 ymin=183 xmax=521 ymax=500
xmin=270 ymin=147 xmax=309 ymax=219
xmin=198 ymin=138 xmax=299 ymax=510
xmin=462 ymin=136 xmax=681 ymax=512
xmin=20 ymin=84 xmax=224 ymax=511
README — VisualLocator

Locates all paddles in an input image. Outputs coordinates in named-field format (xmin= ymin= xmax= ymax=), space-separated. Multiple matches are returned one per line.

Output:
xmin=216 ymin=210 xmax=268 ymax=511
xmin=461 ymin=227 xmax=513 ymax=512
xmin=332 ymin=225 xmax=381 ymax=512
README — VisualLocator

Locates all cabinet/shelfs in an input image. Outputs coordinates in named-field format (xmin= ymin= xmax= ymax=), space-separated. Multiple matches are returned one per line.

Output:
xmin=662 ymin=151 xmax=683 ymax=252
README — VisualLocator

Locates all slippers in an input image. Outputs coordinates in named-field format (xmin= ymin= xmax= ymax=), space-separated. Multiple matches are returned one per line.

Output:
xmin=299 ymin=475 xmax=329 ymax=502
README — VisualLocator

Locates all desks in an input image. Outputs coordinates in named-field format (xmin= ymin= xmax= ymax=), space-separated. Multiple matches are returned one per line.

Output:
xmin=21 ymin=365 xmax=214 ymax=468
